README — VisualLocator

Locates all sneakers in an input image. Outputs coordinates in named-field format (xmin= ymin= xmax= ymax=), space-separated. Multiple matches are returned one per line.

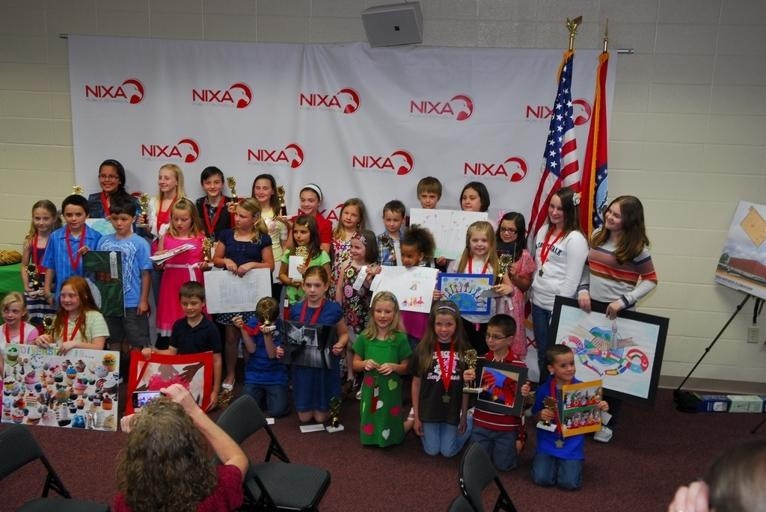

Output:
xmin=594 ymin=426 xmax=615 ymax=443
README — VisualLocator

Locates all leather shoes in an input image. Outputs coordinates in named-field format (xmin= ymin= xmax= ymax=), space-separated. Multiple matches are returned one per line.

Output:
xmin=221 ymin=377 xmax=235 ymax=392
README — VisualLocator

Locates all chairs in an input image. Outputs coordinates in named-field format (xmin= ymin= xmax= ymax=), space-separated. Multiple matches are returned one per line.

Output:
xmin=446 ymin=441 xmax=518 ymax=511
xmin=0 ymin=422 xmax=114 ymax=512
xmin=210 ymin=395 xmax=332 ymax=511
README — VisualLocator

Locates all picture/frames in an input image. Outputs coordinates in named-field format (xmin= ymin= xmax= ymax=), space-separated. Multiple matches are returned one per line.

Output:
xmin=472 ymin=358 xmax=529 ymax=418
xmin=438 ymin=272 xmax=493 ymax=316
xmin=539 ymin=295 xmax=671 ymax=406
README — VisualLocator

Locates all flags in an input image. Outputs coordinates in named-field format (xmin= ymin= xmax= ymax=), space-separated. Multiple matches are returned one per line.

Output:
xmin=526 ymin=15 xmax=581 ymax=249
xmin=580 ymin=17 xmax=609 ymax=251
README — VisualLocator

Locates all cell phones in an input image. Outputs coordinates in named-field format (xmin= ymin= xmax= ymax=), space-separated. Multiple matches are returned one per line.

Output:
xmin=132 ymin=391 xmax=165 ymax=408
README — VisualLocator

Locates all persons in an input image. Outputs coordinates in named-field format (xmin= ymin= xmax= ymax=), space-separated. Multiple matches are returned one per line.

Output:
xmin=0 ymin=158 xmax=658 ymax=489
xmin=111 ymin=383 xmax=250 ymax=511
xmin=666 ymin=438 xmax=765 ymax=511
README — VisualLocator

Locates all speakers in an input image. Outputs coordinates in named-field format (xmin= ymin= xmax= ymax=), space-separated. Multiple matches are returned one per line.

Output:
xmin=360 ymin=2 xmax=424 ymax=49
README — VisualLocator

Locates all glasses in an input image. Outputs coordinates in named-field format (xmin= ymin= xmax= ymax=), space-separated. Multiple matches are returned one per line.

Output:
xmin=499 ymin=228 xmax=518 ymax=232
xmin=98 ymin=174 xmax=118 ymax=180
xmin=486 ymin=332 xmax=503 ymax=340
